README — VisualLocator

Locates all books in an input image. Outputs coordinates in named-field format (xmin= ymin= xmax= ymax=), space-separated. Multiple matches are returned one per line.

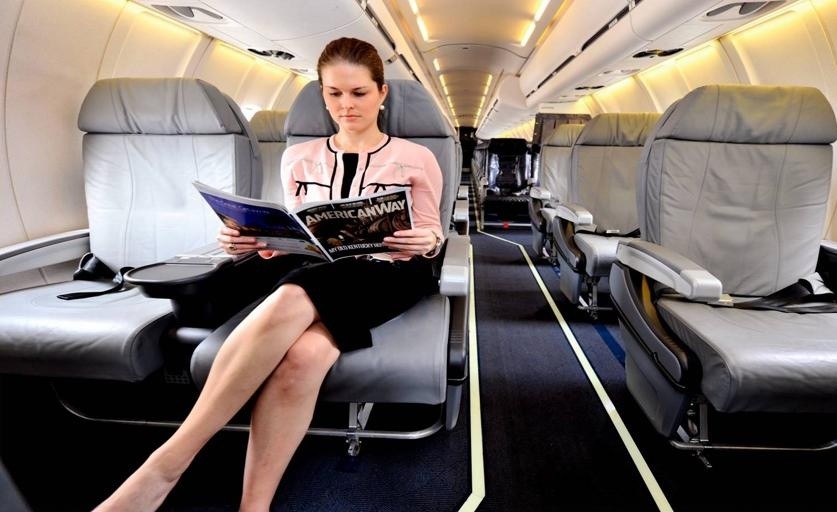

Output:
xmin=187 ymin=179 xmax=414 ymax=265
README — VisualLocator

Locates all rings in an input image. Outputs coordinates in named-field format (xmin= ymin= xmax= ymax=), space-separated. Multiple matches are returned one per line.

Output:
xmin=227 ymin=243 xmax=238 ymax=253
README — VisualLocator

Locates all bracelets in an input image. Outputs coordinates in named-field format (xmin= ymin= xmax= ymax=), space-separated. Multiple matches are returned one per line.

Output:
xmin=422 ymin=229 xmax=441 ymax=258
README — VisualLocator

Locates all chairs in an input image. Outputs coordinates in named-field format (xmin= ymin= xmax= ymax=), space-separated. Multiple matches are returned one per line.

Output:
xmin=471 ymin=136 xmax=541 ymax=234
xmin=0 ymin=78 xmax=470 ymax=457
xmin=527 ymin=113 xmax=663 ymax=319
xmin=609 ymin=84 xmax=837 ymax=468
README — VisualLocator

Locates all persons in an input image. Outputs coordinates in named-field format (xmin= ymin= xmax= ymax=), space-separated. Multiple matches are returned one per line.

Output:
xmin=87 ymin=36 xmax=449 ymax=511
